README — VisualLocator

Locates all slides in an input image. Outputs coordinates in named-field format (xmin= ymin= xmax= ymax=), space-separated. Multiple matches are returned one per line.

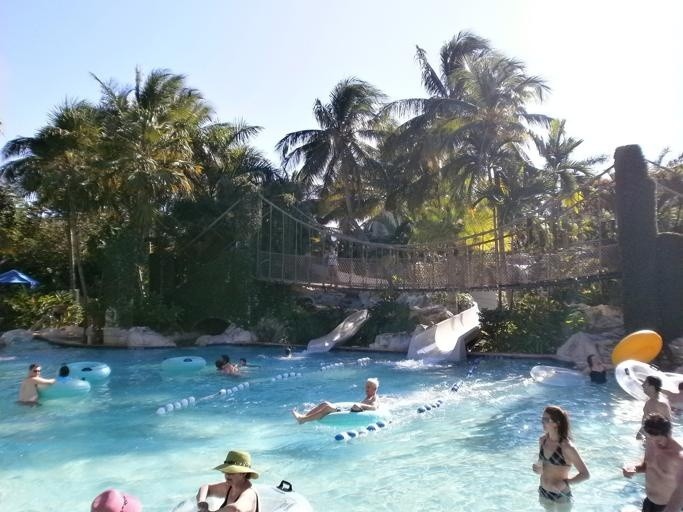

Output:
xmin=306 ymin=259 xmax=500 ymax=368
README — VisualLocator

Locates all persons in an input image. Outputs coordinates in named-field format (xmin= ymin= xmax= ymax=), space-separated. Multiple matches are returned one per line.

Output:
xmin=16 ymin=362 xmax=57 ymax=408
xmin=238 ymin=358 xmax=250 ymax=367
xmin=292 ymin=376 xmax=379 ymax=425
xmin=214 ymin=359 xmax=224 ymax=371
xmin=657 ymin=382 xmax=682 ymax=418
xmin=634 ymin=374 xmax=671 ymax=450
xmin=324 ymin=244 xmax=340 ymax=288
xmin=195 ymin=449 xmax=259 ymax=512
xmin=57 ymin=365 xmax=73 ymax=383
xmin=580 ymin=354 xmax=610 ymax=387
xmin=620 ymin=412 xmax=682 ymax=511
xmin=530 ymin=403 xmax=591 ymax=512
xmin=219 ymin=353 xmax=239 ymax=375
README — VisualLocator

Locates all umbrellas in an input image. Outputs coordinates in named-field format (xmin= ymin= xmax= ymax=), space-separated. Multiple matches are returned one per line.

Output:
xmin=0 ymin=267 xmax=42 ymax=289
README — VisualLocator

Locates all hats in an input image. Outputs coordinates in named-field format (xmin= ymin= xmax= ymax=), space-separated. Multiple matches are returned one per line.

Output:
xmin=646 ymin=376 xmax=663 ymax=386
xmin=212 ymin=450 xmax=260 ymax=481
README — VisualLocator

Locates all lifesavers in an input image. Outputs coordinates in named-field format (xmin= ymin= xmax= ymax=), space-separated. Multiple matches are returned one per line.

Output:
xmin=319 ymin=401 xmax=391 ymax=427
xmin=162 ymin=356 xmax=204 ymax=373
xmin=38 ymin=378 xmax=90 ymax=398
xmin=170 ymin=480 xmax=313 ymax=512
xmin=530 ymin=364 xmax=585 ymax=387
xmin=58 ymin=361 xmax=110 ymax=380
xmin=612 ymin=329 xmax=683 ymax=404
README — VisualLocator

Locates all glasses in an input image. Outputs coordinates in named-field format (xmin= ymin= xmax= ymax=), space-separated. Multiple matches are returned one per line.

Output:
xmin=540 ymin=417 xmax=560 ymax=424
xmin=32 ymin=370 xmax=42 ymax=373
